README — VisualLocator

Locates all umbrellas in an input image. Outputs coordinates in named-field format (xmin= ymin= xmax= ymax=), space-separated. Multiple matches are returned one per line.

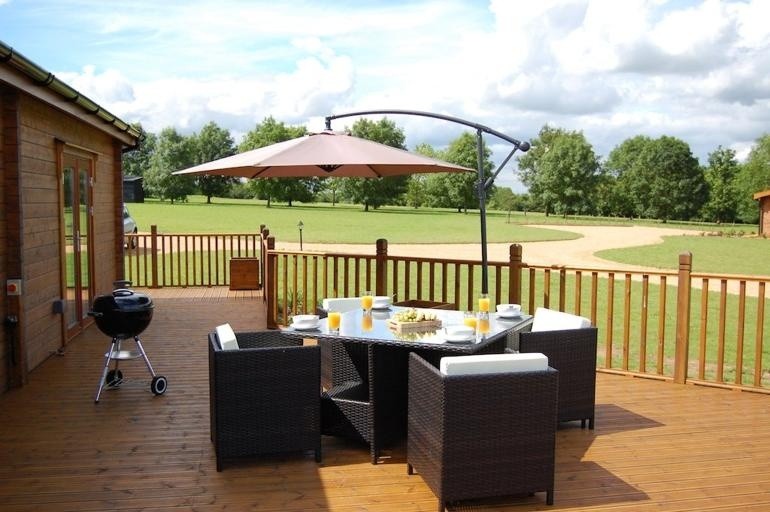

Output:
xmin=174 ymin=109 xmax=533 ymax=293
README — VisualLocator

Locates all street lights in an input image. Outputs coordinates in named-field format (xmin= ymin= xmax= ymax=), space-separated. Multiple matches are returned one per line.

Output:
xmin=296 ymin=219 xmax=305 ymax=251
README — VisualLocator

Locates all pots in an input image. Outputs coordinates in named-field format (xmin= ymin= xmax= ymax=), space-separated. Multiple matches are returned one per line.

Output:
xmin=87 ymin=281 xmax=153 ymax=337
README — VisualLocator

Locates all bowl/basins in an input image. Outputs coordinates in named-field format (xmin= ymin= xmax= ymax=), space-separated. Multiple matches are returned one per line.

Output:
xmin=293 ymin=314 xmax=320 ymax=327
xmin=496 ymin=303 xmax=523 ymax=313
xmin=372 ymin=295 xmax=392 ymax=306
xmin=445 ymin=326 xmax=475 ymax=336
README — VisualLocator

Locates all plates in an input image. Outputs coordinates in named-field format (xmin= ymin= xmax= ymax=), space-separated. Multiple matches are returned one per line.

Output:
xmin=290 ymin=323 xmax=320 ymax=330
xmin=372 ymin=305 xmax=390 ymax=310
xmin=496 ymin=312 xmax=525 ymax=318
xmin=443 ymin=335 xmax=478 ymax=341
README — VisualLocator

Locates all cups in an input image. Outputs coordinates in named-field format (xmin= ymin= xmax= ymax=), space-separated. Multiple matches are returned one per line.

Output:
xmin=464 ymin=292 xmax=491 ymax=334
xmin=360 ymin=291 xmax=373 ymax=314
xmin=361 ymin=312 xmax=373 ymax=331
xmin=328 ymin=311 xmax=341 ymax=333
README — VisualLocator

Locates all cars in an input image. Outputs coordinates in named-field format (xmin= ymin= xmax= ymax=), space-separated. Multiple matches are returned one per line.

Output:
xmin=123 ymin=201 xmax=140 ymax=250
